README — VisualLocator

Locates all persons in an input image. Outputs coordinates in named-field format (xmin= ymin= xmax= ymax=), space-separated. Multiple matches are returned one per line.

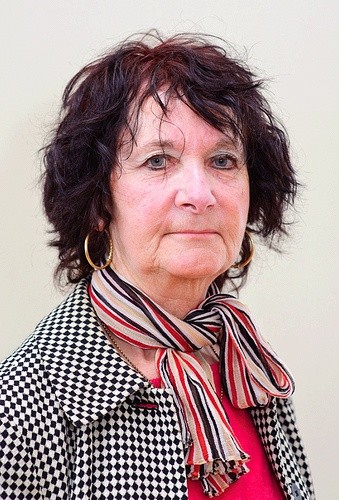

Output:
xmin=1 ymin=36 xmax=314 ymax=500
